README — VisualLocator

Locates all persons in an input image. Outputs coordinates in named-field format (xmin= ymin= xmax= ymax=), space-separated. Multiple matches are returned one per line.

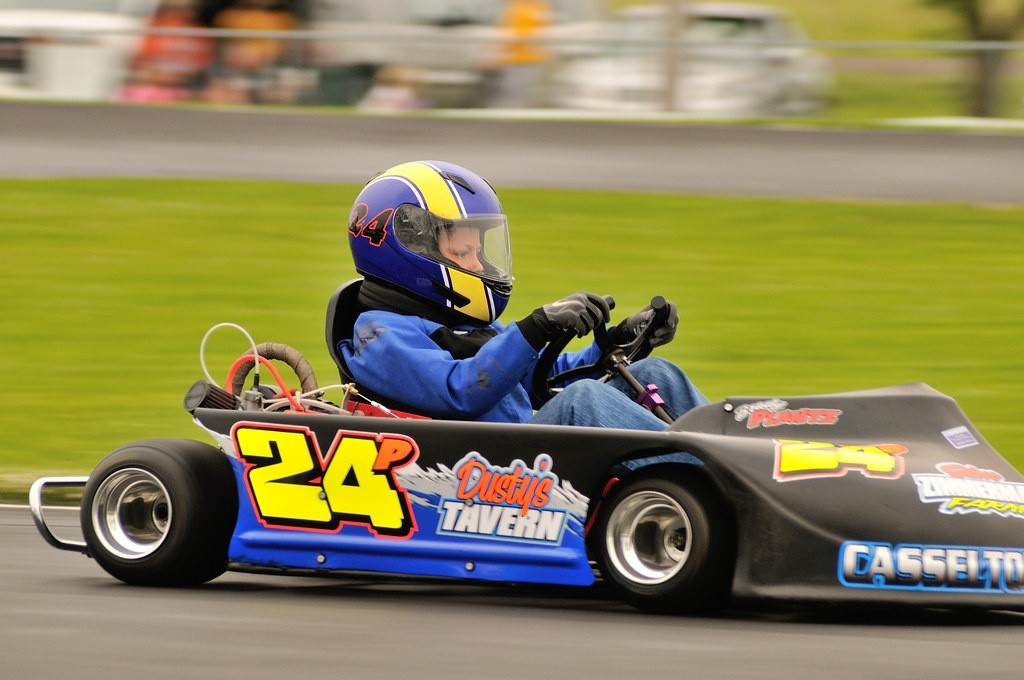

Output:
xmin=349 ymin=161 xmax=712 ymax=432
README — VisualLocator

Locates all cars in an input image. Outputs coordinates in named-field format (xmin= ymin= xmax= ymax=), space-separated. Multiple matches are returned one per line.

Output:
xmin=538 ymin=4 xmax=843 ymax=116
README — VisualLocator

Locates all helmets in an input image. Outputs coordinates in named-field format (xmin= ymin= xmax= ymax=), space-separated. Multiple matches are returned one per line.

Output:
xmin=348 ymin=160 xmax=513 ymax=325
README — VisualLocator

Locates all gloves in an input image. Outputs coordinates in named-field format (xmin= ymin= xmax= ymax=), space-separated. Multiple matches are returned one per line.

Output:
xmin=532 ymin=291 xmax=610 ymax=339
xmin=621 ymin=300 xmax=680 ymax=349
